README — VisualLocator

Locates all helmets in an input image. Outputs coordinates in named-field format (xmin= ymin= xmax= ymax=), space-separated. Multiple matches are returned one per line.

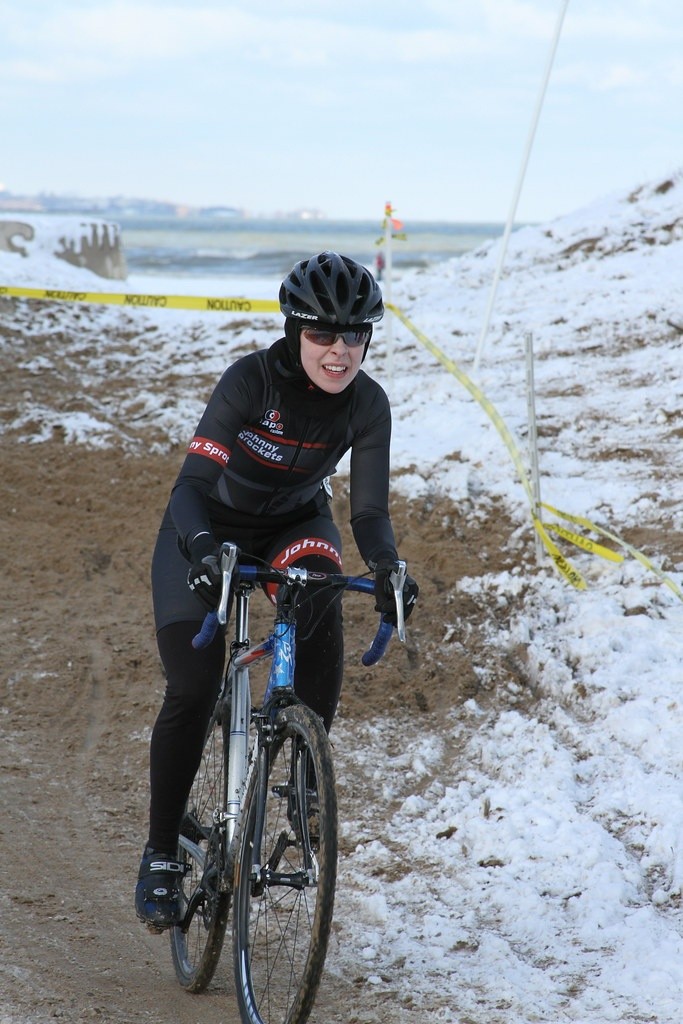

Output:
xmin=279 ymin=251 xmax=384 ymax=324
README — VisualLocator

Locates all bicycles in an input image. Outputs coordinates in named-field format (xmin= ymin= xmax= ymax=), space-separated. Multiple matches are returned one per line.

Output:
xmin=142 ymin=542 xmax=410 ymax=1024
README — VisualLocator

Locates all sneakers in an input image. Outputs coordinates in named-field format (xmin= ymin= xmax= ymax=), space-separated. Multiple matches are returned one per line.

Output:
xmin=271 ymin=780 xmax=320 ymax=852
xmin=135 ymin=848 xmax=191 ymax=926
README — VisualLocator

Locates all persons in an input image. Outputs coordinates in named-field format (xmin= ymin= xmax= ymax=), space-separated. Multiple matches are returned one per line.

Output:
xmin=133 ymin=249 xmax=422 ymax=927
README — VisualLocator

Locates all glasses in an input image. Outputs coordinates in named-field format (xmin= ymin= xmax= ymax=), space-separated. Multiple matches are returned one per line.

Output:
xmin=300 ymin=325 xmax=371 ymax=347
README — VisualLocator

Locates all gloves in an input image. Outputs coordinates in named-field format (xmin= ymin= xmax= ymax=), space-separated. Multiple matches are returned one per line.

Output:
xmin=187 ymin=533 xmax=223 ymax=613
xmin=374 ymin=560 xmax=419 ymax=629
